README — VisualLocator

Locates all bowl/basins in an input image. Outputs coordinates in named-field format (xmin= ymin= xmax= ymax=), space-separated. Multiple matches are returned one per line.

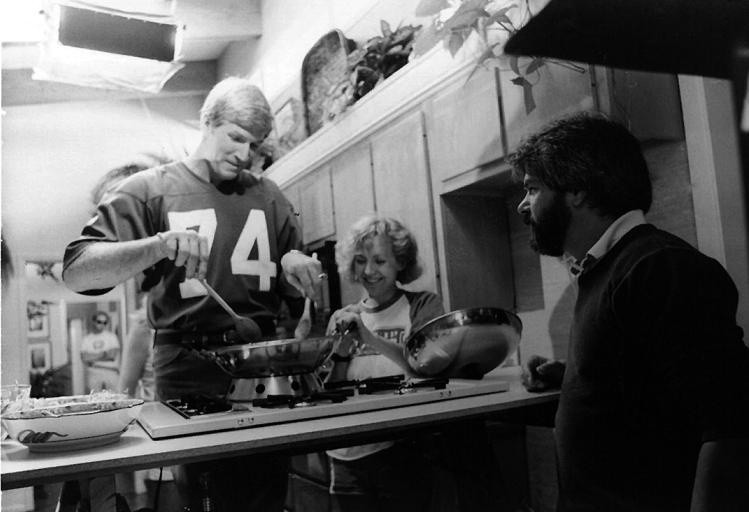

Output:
xmin=4 ymin=393 xmax=145 ymax=454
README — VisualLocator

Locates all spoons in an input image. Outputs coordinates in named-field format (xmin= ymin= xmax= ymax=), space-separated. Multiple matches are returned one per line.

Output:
xmin=294 ymin=252 xmax=318 ymax=341
xmin=156 ymin=232 xmax=262 ymax=345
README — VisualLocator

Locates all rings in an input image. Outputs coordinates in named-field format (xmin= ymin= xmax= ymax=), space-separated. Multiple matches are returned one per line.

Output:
xmin=317 ymin=272 xmax=328 ymax=280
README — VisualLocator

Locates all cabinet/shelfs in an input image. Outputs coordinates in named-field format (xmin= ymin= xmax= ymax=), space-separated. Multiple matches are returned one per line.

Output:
xmin=260 ymin=0 xmax=684 ymax=366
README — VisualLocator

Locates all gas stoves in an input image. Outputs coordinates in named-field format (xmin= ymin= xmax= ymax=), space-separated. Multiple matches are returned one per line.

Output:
xmin=132 ymin=372 xmax=509 ymax=442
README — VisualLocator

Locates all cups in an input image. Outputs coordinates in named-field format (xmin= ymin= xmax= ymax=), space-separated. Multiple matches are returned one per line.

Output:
xmin=1 ymin=383 xmax=32 ymax=402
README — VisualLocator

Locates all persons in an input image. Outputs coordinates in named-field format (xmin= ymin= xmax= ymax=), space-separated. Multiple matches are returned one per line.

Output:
xmin=504 ymin=106 xmax=748 ymax=512
xmin=62 ymin=73 xmax=327 ymax=512
xmin=119 ymin=282 xmax=187 ymax=512
xmin=80 ymin=312 xmax=121 ymax=393
xmin=325 ymin=213 xmax=447 ymax=512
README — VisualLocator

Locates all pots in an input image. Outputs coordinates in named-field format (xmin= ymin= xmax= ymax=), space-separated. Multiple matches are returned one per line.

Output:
xmin=205 ymin=336 xmax=333 ymax=376
xmin=403 ymin=306 xmax=523 ymax=378
